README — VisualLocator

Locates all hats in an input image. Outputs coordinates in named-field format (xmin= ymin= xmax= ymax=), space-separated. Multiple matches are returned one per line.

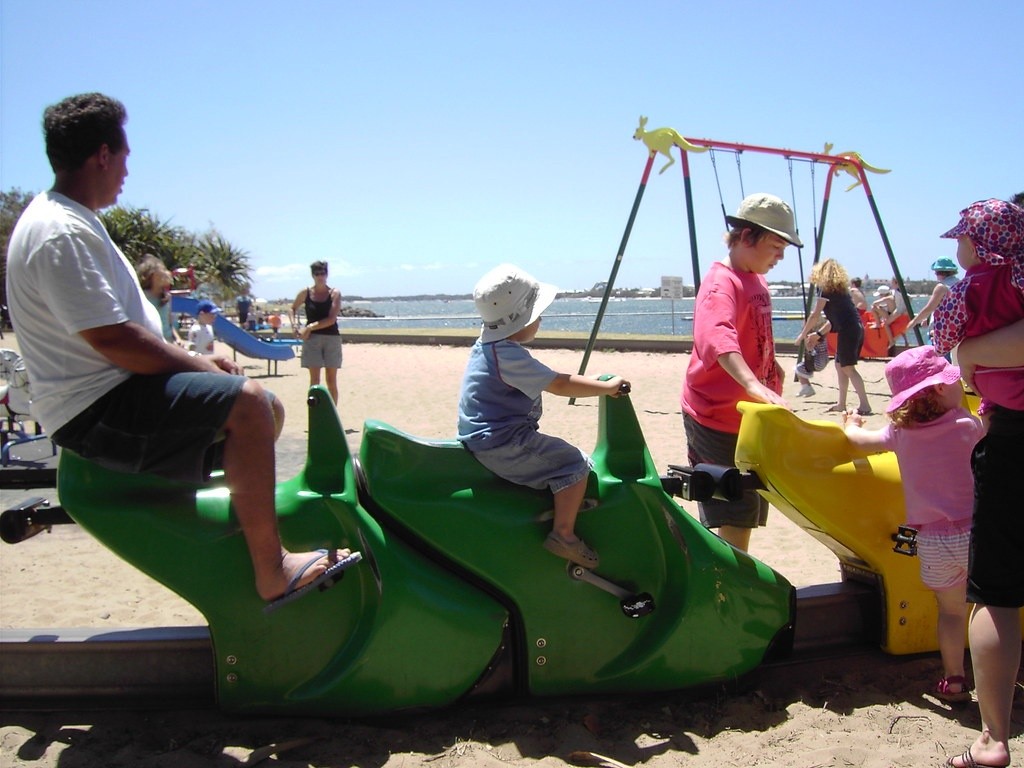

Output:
xmin=726 ymin=199 xmax=804 ymax=248
xmin=196 ymin=299 xmax=223 ymax=314
xmin=940 ymin=197 xmax=1024 ymax=293
xmin=873 ymin=285 xmax=889 ymax=297
xmin=472 ymin=269 xmax=560 ymax=346
xmin=932 ymin=258 xmax=957 ymax=275
xmin=882 ymin=343 xmax=961 ymax=415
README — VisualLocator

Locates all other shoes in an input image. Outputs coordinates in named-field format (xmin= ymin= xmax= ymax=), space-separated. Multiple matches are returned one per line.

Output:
xmin=797 ymin=384 xmax=815 ymax=397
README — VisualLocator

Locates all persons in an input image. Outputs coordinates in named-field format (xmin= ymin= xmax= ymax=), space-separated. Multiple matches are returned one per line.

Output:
xmin=287 ymin=262 xmax=342 ymax=407
xmin=847 ymin=270 xmax=867 ymax=316
xmin=6 ymin=93 xmax=362 ymax=616
xmin=187 ymin=302 xmax=223 ymax=354
xmin=235 ymin=286 xmax=254 ymax=329
xmin=456 ymin=262 xmax=631 ymax=568
xmin=795 ymin=328 xmax=828 ymax=398
xmin=247 ymin=303 xmax=282 ymax=333
xmin=681 ymin=193 xmax=805 ymax=553
xmin=870 ymin=277 xmax=906 ymax=351
xmin=134 ymin=254 xmax=184 ymax=350
xmin=928 ymin=198 xmax=1024 ymax=768
xmin=793 ymin=258 xmax=875 ymax=418
xmin=906 ymin=256 xmax=961 ymax=357
xmin=840 ymin=344 xmax=990 ymax=702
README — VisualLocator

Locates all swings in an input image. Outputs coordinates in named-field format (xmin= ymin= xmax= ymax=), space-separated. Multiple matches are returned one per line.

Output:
xmin=705 ymin=148 xmax=745 ymax=232
xmin=785 ymin=159 xmax=820 ymax=372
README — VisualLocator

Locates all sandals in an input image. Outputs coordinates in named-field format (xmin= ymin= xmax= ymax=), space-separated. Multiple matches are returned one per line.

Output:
xmin=935 ymin=672 xmax=971 ymax=703
xmin=543 ymin=531 xmax=600 ymax=568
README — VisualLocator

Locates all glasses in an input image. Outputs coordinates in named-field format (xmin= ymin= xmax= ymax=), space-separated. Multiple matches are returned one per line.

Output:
xmin=312 ymin=271 xmax=326 ymax=276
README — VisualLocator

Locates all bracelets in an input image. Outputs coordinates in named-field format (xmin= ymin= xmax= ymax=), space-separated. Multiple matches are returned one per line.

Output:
xmin=309 ymin=322 xmax=315 ymax=332
xmin=816 ymin=330 xmax=823 ymax=338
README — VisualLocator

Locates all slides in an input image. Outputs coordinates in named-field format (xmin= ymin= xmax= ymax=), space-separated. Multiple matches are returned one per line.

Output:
xmin=171 ymin=295 xmax=295 ymax=361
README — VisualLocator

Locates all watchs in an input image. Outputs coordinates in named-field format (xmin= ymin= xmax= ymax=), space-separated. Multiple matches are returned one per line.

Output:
xmin=187 ymin=350 xmax=201 ymax=358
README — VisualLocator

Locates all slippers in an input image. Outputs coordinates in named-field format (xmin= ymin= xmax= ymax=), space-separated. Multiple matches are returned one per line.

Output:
xmin=1011 ymin=667 xmax=1024 ymax=709
xmin=946 ymin=745 xmax=1007 ymax=768
xmin=261 ymin=548 xmax=364 ymax=616
xmin=825 ymin=406 xmax=845 ymax=412
xmin=849 ymin=408 xmax=873 ymax=415
